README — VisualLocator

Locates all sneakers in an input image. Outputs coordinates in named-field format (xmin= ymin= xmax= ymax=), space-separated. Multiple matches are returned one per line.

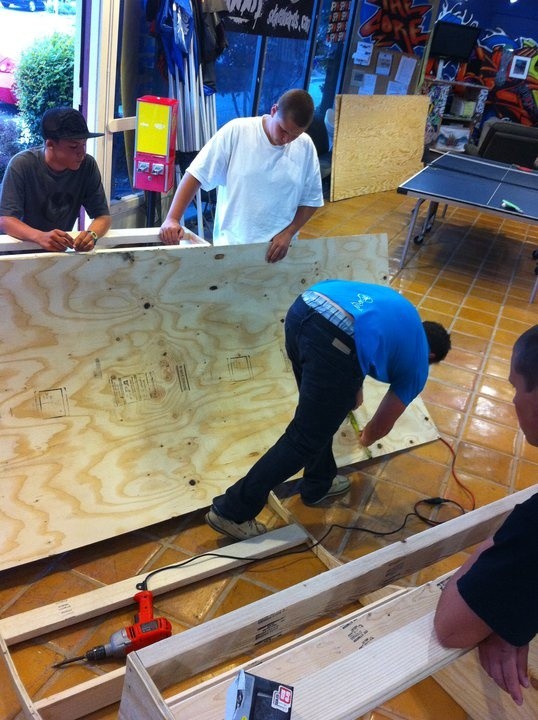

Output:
xmin=302 ymin=474 xmax=351 ymax=504
xmin=205 ymin=512 xmax=266 ymax=540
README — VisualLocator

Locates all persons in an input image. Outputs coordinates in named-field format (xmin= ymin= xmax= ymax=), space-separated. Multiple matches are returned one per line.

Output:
xmin=434 ymin=323 xmax=538 ymax=706
xmin=0 ymin=108 xmax=111 ymax=252
xmin=203 ymin=280 xmax=452 ymax=542
xmin=159 ymin=90 xmax=325 ymax=264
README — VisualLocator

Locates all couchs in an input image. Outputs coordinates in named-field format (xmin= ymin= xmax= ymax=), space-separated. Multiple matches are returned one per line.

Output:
xmin=464 ymin=119 xmax=538 ymax=168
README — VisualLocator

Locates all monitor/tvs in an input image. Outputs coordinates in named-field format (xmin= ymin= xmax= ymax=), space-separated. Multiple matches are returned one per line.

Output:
xmin=427 ymin=21 xmax=481 ymax=62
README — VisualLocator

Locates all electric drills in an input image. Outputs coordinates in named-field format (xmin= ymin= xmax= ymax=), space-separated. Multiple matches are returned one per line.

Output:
xmin=52 ymin=590 xmax=171 ymax=667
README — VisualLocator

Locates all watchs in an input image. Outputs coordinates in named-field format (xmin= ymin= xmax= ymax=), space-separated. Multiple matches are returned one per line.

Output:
xmin=85 ymin=231 xmax=98 ymax=245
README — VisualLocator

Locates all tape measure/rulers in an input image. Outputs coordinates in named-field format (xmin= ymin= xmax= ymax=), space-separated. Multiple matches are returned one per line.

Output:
xmin=349 ymin=411 xmax=372 ymax=459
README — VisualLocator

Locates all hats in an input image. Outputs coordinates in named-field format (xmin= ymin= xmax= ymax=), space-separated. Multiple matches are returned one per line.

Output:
xmin=41 ymin=106 xmax=105 ymax=140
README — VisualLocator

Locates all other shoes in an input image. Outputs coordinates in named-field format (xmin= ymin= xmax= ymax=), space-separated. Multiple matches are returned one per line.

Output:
xmin=463 ymin=144 xmax=481 ymax=157
